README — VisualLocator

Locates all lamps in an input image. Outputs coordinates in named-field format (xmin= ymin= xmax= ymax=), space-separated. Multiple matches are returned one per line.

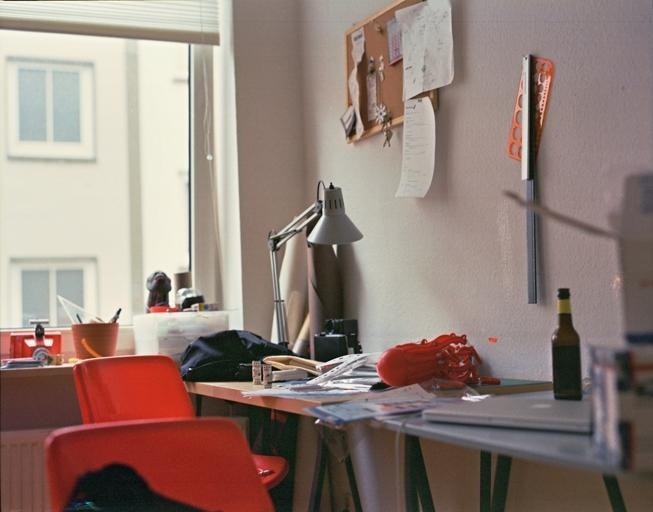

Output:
xmin=267 ymin=180 xmax=364 ymax=350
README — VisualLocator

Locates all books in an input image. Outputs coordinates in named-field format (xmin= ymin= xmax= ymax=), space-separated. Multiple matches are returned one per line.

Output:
xmin=419 ymin=394 xmax=592 ymax=437
xmin=318 ymin=394 xmax=432 ymax=422
xmin=305 ymin=407 xmax=341 ymax=424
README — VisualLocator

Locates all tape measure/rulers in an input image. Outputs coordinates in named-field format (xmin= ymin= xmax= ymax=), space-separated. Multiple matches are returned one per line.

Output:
xmin=57 ymin=294 xmax=104 ymax=324
xmin=508 ymin=54 xmax=554 ymax=304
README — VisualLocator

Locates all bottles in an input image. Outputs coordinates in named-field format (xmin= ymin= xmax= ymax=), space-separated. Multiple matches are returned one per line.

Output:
xmin=551 ymin=287 xmax=582 ymax=401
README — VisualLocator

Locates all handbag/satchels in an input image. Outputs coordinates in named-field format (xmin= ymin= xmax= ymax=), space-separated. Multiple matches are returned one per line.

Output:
xmin=177 ymin=329 xmax=293 ymax=382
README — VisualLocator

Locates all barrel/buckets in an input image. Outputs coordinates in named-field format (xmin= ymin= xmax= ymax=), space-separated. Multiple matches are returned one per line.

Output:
xmin=71 ymin=322 xmax=118 ymax=361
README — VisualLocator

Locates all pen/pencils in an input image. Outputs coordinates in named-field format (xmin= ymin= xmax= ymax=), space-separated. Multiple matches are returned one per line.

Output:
xmin=77 ymin=308 xmax=121 ymax=323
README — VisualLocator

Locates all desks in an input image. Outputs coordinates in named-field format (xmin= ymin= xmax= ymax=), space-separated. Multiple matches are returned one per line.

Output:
xmin=184 ymin=380 xmax=554 ymax=512
xmin=363 ymin=391 xmax=653 ymax=512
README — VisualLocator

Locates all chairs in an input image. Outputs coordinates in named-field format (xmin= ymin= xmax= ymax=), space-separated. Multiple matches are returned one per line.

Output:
xmin=73 ymin=355 xmax=290 ymax=492
xmin=43 ymin=414 xmax=277 ymax=512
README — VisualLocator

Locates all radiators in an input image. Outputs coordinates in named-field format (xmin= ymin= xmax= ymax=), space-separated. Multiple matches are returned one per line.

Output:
xmin=0 ymin=428 xmax=51 ymax=512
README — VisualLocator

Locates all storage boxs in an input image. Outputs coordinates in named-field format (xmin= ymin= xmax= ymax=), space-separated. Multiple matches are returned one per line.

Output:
xmin=133 ymin=311 xmax=229 ymax=360
